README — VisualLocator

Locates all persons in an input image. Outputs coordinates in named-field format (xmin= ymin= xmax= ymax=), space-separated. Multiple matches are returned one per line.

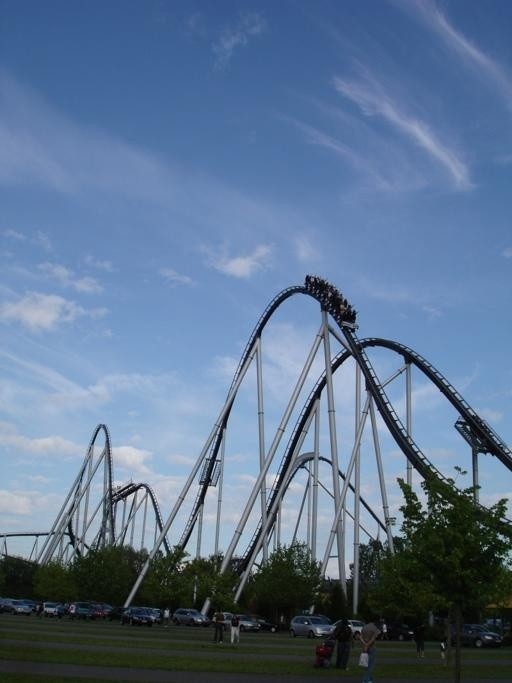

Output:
xmin=381 ymin=622 xmax=390 ymax=640
xmin=209 ymin=608 xmax=227 ymax=643
xmin=441 ymin=636 xmax=448 ymax=667
xmin=230 ymin=614 xmax=240 ymax=644
xmin=360 ymin=617 xmax=381 ymax=683
xmin=414 ymin=624 xmax=426 ymax=658
xmin=54 ymin=602 xmax=65 ymax=625
xmin=332 ymin=618 xmax=355 ymax=670
xmin=67 ymin=602 xmax=77 ymax=621
xmin=163 ymin=607 xmax=170 ymax=625
xmin=305 ymin=275 xmax=357 ymax=320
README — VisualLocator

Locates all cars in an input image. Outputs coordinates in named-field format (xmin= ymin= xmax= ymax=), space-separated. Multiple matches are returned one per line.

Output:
xmin=289 ymin=616 xmax=416 ymax=641
xmin=444 ymin=623 xmax=504 ymax=648
xmin=0 ymin=598 xmax=279 ymax=633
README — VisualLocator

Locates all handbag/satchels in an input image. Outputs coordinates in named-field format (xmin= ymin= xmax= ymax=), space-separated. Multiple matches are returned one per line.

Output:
xmin=357 ymin=652 xmax=370 ymax=667
